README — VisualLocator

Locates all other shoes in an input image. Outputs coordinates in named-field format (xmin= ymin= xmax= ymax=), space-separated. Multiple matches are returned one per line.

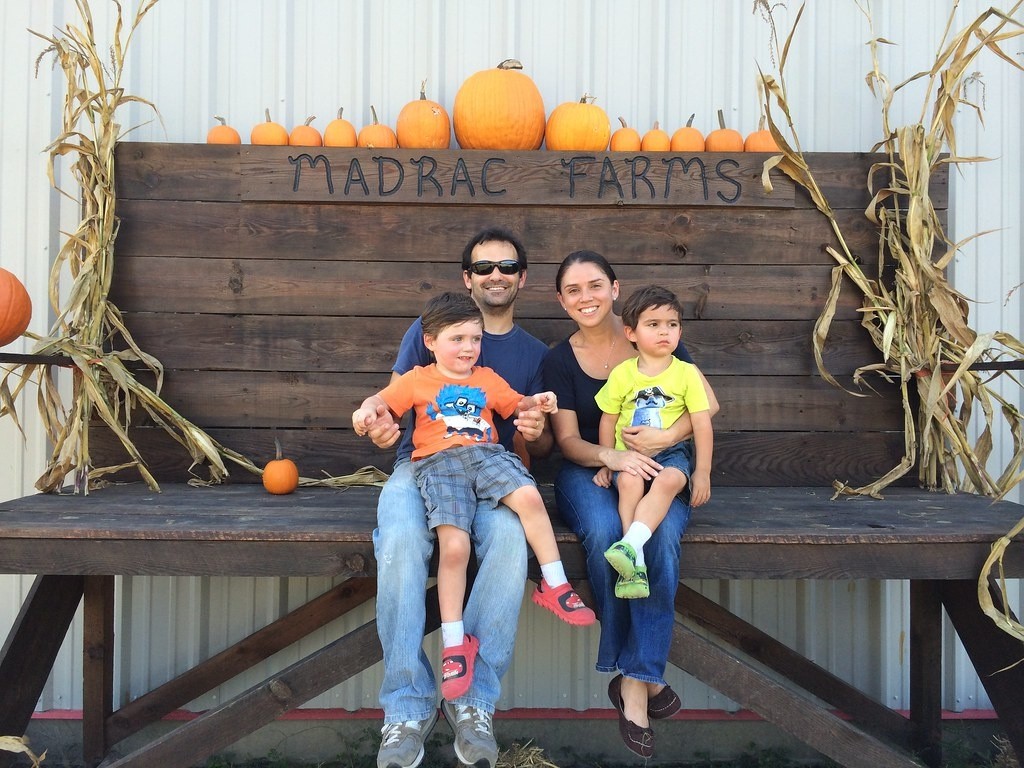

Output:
xmin=607 ymin=674 xmax=655 ymax=759
xmin=646 ymin=681 xmax=681 ymax=719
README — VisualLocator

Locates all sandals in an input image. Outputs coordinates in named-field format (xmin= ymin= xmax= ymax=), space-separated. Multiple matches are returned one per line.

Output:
xmin=614 ymin=563 xmax=649 ymax=599
xmin=441 ymin=633 xmax=479 ymax=701
xmin=604 ymin=541 xmax=637 ymax=580
xmin=531 ymin=578 xmax=596 ymax=626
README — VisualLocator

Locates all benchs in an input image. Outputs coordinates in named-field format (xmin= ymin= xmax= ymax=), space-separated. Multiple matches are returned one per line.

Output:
xmin=1 ymin=141 xmax=1023 ymax=768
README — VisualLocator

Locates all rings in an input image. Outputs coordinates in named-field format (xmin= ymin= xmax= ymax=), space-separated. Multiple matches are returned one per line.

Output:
xmin=535 ymin=421 xmax=539 ymax=428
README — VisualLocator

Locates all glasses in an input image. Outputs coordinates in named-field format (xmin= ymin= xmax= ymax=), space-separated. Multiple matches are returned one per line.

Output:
xmin=467 ymin=259 xmax=523 ymax=275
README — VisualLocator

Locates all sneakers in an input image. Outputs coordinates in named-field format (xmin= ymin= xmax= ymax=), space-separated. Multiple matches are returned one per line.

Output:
xmin=377 ymin=707 xmax=438 ymax=768
xmin=440 ymin=695 xmax=498 ymax=768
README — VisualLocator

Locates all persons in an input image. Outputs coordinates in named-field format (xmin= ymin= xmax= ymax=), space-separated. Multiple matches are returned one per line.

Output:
xmin=352 ymin=292 xmax=594 ymax=701
xmin=591 ymin=285 xmax=714 ymax=598
xmin=372 ymin=225 xmax=555 ymax=768
xmin=542 ymin=249 xmax=719 ymax=759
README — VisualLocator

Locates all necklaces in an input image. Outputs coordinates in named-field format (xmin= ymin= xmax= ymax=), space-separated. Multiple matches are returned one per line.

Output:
xmin=581 ymin=323 xmax=619 ymax=369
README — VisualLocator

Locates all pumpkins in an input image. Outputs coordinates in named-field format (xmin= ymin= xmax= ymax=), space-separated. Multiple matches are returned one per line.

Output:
xmin=250 ymin=108 xmax=322 ymax=147
xmin=263 ymin=439 xmax=298 ymax=494
xmin=453 ymin=59 xmax=611 ymax=152
xmin=611 ymin=110 xmax=783 ymax=153
xmin=205 ymin=116 xmax=241 ymax=144
xmin=0 ymin=267 xmax=32 ymax=348
xmin=322 ymin=82 xmax=450 ymax=150
